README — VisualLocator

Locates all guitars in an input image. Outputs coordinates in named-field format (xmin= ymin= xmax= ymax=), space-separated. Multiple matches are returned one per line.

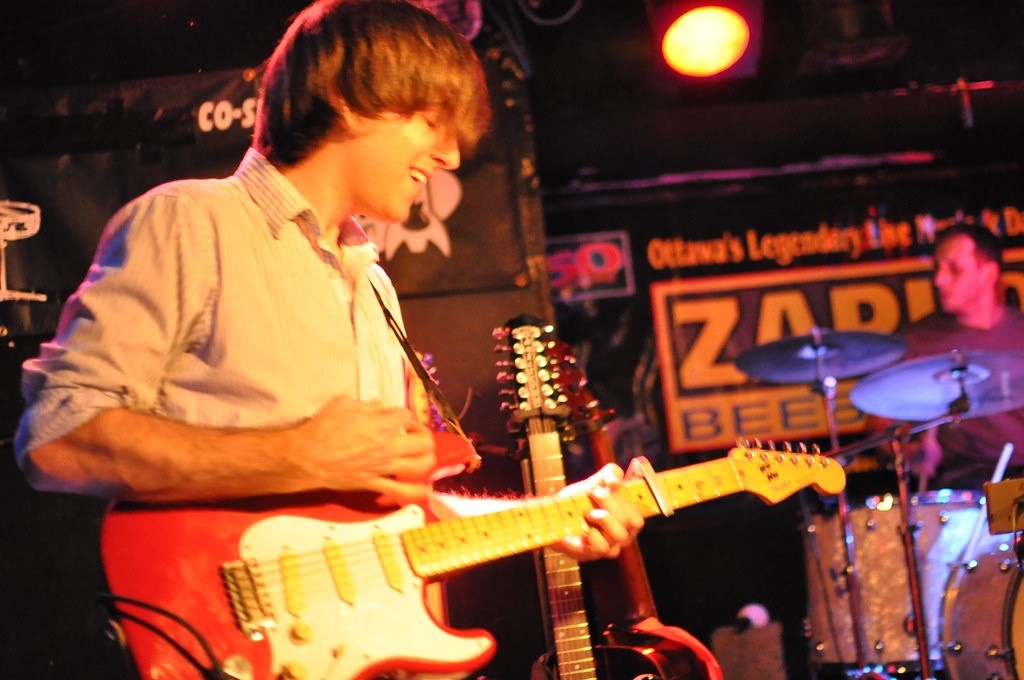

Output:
xmin=491 ymin=311 xmax=671 ymax=679
xmin=87 ymin=435 xmax=848 ymax=680
xmin=547 ymin=338 xmax=726 ymax=680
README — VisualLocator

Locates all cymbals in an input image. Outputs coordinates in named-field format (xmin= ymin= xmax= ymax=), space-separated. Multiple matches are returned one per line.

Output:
xmin=848 ymin=345 xmax=1024 ymax=424
xmin=732 ymin=328 xmax=909 ymax=387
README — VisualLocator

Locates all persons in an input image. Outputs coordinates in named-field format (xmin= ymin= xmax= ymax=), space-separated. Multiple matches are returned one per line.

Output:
xmin=868 ymin=226 xmax=1020 ymax=476
xmin=13 ymin=1 xmax=644 ymax=680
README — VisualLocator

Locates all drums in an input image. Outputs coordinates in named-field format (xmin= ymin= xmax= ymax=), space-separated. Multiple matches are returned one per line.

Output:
xmin=938 ymin=516 xmax=1024 ymax=680
xmin=800 ymin=486 xmax=997 ymax=674
